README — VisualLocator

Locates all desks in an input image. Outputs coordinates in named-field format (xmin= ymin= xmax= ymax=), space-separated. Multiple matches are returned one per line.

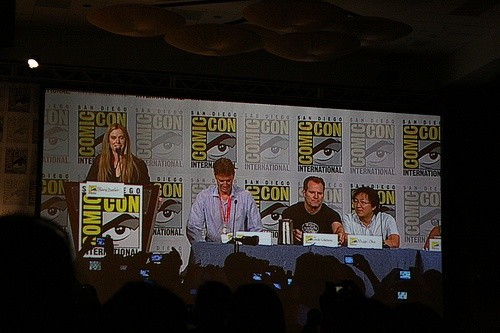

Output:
xmin=189 ymin=240 xmax=442 ymax=297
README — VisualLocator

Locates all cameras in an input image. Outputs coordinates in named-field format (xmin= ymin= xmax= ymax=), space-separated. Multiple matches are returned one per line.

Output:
xmin=140 ymin=269 xmax=150 ymax=278
xmin=152 ymin=254 xmax=166 ymax=262
xmin=344 ymin=255 xmax=356 ymax=264
xmin=96 ymin=237 xmax=106 ymax=247
xmin=397 ymin=291 xmax=408 ymax=300
xmin=398 ymin=270 xmax=412 ymax=280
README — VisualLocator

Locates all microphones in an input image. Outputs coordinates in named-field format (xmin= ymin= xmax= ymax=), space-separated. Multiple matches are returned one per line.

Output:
xmin=380 ymin=204 xmax=388 ymax=246
xmin=232 ymin=200 xmax=237 ymax=244
xmin=116 ymin=147 xmax=123 ymax=183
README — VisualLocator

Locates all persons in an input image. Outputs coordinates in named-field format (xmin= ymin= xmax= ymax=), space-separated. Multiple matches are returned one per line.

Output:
xmin=186 ymin=157 xmax=262 ymax=245
xmin=282 ymin=176 xmax=345 ymax=246
xmin=83 ymin=123 xmax=150 ymax=185
xmin=340 ymin=187 xmax=400 ymax=248
xmin=0 ymin=211 xmax=500 ymax=333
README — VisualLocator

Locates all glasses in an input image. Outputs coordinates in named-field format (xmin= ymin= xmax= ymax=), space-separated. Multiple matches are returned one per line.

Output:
xmin=354 ymin=200 xmax=370 ymax=205
